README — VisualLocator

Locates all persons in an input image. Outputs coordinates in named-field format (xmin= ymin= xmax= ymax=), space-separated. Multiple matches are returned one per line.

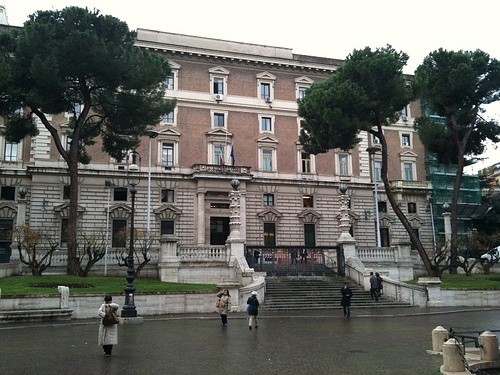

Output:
xmin=247 ymin=290 xmax=260 ymax=330
xmin=253 ymin=247 xmax=261 ymax=264
xmin=291 ymin=247 xmax=308 ymax=264
xmin=216 ymin=289 xmax=232 ymax=327
xmin=369 ymin=271 xmax=383 ymax=298
xmin=97 ymin=296 xmax=119 ymax=356
xmin=340 ymin=282 xmax=352 ymax=317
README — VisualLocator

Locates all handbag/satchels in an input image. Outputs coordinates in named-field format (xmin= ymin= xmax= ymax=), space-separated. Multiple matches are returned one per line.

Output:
xmin=102 ymin=307 xmax=119 ymax=326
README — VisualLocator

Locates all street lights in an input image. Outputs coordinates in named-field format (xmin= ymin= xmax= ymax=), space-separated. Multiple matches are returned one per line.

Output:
xmin=366 ymin=147 xmax=381 ymax=247
xmin=121 ymin=151 xmax=142 ymax=317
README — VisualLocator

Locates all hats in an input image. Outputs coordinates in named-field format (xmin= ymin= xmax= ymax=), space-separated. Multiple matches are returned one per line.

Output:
xmin=251 ymin=290 xmax=257 ymax=295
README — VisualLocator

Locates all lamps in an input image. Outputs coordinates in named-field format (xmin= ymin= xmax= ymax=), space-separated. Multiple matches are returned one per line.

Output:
xmin=231 ymin=177 xmax=240 ymax=191
xmin=338 ymin=181 xmax=348 ymax=195
xmin=18 ymin=187 xmax=27 ymax=199
xmin=443 ymin=202 xmax=450 ymax=211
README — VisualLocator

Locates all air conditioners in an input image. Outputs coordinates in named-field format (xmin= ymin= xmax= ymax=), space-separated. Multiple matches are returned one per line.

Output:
xmin=214 ymin=94 xmax=223 ymax=101
xmin=266 ymin=97 xmax=272 ymax=103
xmin=402 ymin=116 xmax=408 ymax=122
